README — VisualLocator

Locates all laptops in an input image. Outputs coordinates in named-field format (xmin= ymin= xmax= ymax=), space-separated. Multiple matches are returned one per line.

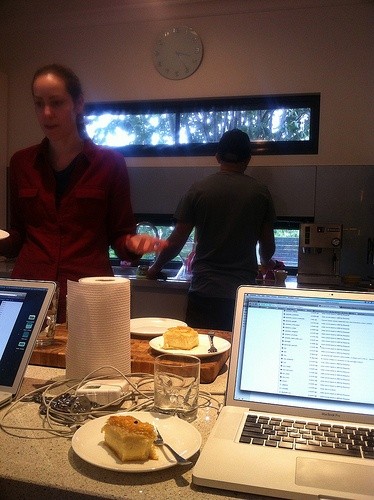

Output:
xmin=0 ymin=277 xmax=56 ymax=407
xmin=192 ymin=282 xmax=374 ymax=500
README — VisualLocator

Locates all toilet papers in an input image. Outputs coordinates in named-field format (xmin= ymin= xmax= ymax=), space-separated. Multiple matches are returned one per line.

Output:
xmin=64 ymin=275 xmax=131 ymax=389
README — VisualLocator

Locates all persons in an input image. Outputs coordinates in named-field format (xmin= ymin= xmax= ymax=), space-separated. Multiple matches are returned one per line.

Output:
xmin=0 ymin=64 xmax=169 ymax=325
xmin=148 ymin=129 xmax=276 ymax=332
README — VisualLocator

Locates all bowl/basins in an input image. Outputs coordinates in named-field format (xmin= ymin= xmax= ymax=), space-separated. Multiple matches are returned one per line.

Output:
xmin=255 ymin=269 xmax=288 ymax=282
xmin=343 ymin=275 xmax=361 ymax=284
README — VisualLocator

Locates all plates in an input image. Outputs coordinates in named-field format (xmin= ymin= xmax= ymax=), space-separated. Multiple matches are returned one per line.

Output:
xmin=72 ymin=412 xmax=202 ymax=473
xmin=130 ymin=317 xmax=187 ymax=336
xmin=0 ymin=229 xmax=9 ymax=240
xmin=149 ymin=333 xmax=232 ymax=358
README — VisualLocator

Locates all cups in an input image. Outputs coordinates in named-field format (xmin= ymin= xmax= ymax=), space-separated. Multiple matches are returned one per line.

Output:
xmin=154 ymin=353 xmax=201 ymax=423
xmin=34 ymin=283 xmax=59 ymax=348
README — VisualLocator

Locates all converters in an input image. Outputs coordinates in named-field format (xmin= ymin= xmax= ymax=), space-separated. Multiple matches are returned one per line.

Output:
xmin=78 ymin=379 xmax=130 ymax=405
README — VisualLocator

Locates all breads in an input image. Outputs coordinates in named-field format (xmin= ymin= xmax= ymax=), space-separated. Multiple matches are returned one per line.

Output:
xmin=161 ymin=326 xmax=199 ymax=350
xmin=101 ymin=416 xmax=158 ymax=461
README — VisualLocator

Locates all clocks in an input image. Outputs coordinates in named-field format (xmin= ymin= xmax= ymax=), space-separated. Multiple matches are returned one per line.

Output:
xmin=151 ymin=23 xmax=205 ymax=79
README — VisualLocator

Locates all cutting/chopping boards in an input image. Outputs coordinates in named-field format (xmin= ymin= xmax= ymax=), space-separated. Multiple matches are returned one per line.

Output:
xmin=29 ymin=323 xmax=232 ymax=384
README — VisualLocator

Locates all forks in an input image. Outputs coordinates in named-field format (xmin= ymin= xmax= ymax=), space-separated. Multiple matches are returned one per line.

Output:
xmin=207 ymin=331 xmax=217 ymax=353
xmin=150 ymin=429 xmax=192 ymax=465
